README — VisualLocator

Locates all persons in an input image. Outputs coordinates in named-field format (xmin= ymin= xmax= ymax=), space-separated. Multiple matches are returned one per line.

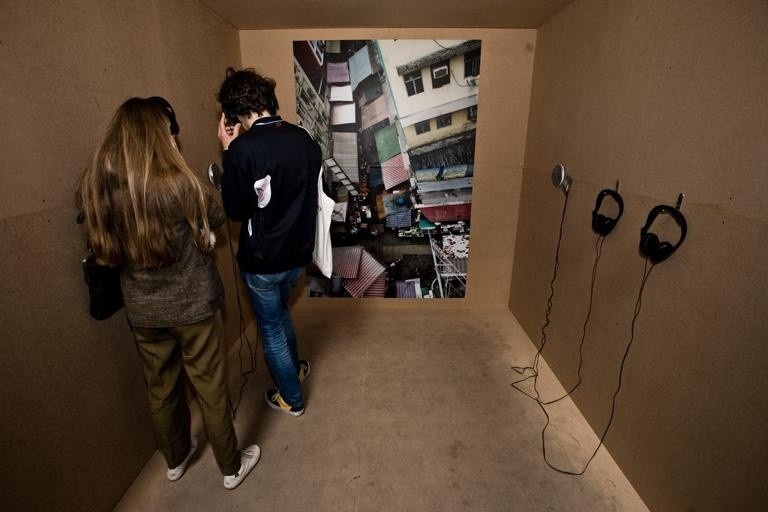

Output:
xmin=215 ymin=66 xmax=323 ymax=416
xmin=74 ymin=96 xmax=264 ymax=490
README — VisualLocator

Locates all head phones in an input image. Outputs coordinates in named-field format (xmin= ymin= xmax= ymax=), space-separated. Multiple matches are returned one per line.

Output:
xmin=220 ymin=72 xmax=279 ymax=124
xmin=143 ymin=96 xmax=182 ymax=152
xmin=639 ymin=204 xmax=687 ymax=264
xmin=591 ymin=189 xmax=624 ymax=236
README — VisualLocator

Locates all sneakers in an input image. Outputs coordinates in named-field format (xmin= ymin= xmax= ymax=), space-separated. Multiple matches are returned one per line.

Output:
xmin=166 ymin=436 xmax=200 ymax=482
xmin=295 ymin=359 xmax=311 ymax=384
xmin=264 ymin=386 xmax=305 ymax=419
xmin=222 ymin=444 xmax=263 ymax=491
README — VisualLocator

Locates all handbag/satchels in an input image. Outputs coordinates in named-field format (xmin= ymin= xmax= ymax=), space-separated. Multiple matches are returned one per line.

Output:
xmin=81 ymin=251 xmax=126 ymax=321
xmin=297 ymin=125 xmax=338 ymax=282
